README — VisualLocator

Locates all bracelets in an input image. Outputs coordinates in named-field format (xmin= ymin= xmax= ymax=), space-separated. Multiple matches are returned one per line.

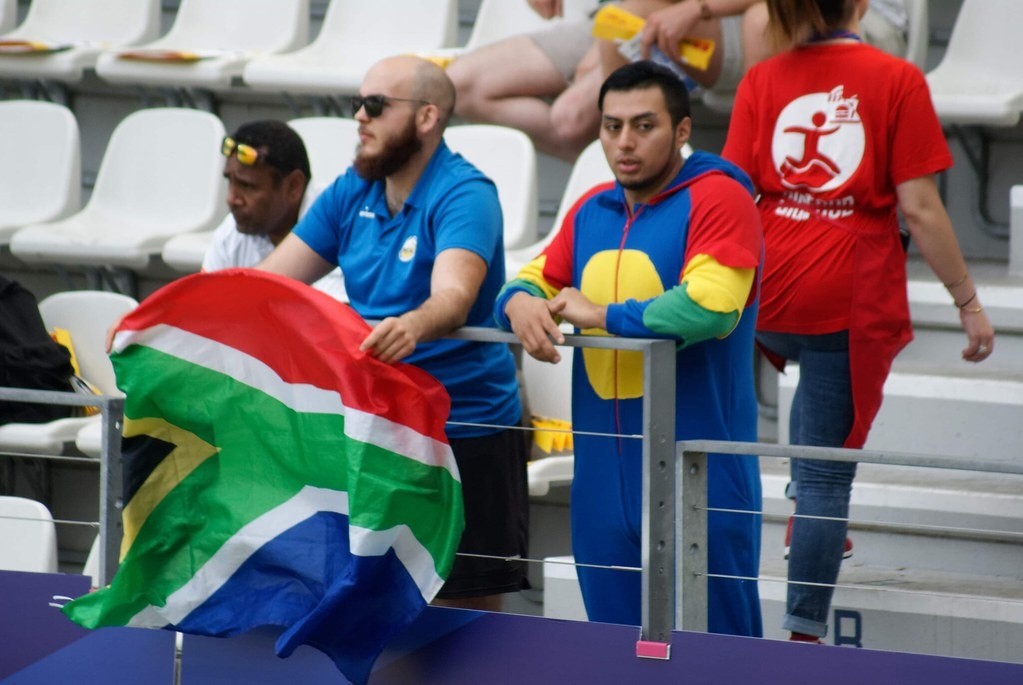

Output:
xmin=954 ymin=293 xmax=984 ymax=313
xmin=945 ymin=272 xmax=970 ymax=289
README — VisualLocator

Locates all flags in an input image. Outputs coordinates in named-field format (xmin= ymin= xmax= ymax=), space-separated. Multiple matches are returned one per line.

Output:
xmin=58 ymin=269 xmax=466 ymax=685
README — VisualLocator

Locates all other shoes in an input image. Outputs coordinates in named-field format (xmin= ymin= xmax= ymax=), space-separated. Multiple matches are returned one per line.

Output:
xmin=784 ymin=513 xmax=854 ymax=559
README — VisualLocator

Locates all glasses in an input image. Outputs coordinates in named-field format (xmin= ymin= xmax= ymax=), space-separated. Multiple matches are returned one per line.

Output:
xmin=352 ymin=96 xmax=430 ymax=118
xmin=221 ymin=137 xmax=294 ymax=171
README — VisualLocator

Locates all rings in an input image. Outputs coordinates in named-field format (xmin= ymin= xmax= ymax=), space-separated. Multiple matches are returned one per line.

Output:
xmin=979 ymin=345 xmax=987 ymax=354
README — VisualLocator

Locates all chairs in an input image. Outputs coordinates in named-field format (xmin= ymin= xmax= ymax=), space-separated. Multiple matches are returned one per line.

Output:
xmin=0 ymin=0 xmax=1022 ymax=502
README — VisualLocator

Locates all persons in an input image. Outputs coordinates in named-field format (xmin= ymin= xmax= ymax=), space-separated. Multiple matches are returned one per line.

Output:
xmin=108 ymin=55 xmax=532 ymax=610
xmin=201 ymin=121 xmax=350 ymax=306
xmin=444 ymin=0 xmax=912 ymax=165
xmin=720 ymin=0 xmax=994 ymax=646
xmin=0 ymin=275 xmax=85 ymax=509
xmin=493 ymin=60 xmax=764 ymax=637
